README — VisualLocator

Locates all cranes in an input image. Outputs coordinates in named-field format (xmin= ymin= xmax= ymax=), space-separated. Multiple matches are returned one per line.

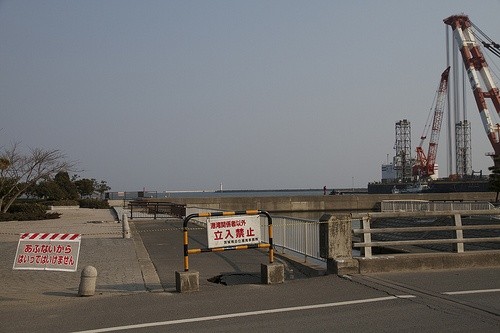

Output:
xmin=410 ymin=65 xmax=451 ymax=183
